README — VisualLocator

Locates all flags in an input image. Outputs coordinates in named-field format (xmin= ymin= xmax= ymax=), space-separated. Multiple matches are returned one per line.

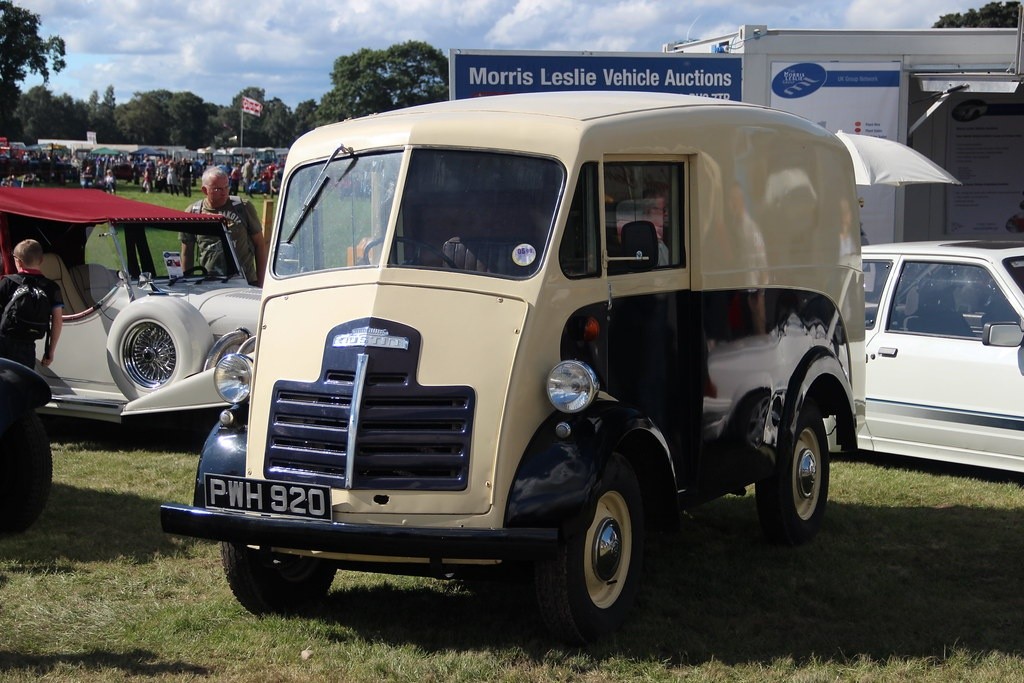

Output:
xmin=242 ymin=95 xmax=263 ymax=116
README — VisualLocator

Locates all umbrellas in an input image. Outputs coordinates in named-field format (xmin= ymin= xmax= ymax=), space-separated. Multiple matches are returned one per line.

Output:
xmin=835 ymin=128 xmax=963 ymax=186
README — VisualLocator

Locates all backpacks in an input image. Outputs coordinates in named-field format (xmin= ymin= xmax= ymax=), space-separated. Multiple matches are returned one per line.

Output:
xmin=0 ymin=274 xmax=51 ymax=341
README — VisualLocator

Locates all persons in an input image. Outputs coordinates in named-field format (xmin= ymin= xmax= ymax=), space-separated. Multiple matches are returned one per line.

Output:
xmin=1 ymin=238 xmax=66 ymax=375
xmin=1 ymin=155 xmax=372 ymax=201
xmin=706 ymin=167 xmax=859 ymax=353
xmin=174 ymin=167 xmax=273 ymax=288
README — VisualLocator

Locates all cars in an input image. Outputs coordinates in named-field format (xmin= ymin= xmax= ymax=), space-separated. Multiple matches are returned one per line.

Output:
xmin=0 ymin=137 xmax=289 ymax=194
xmin=825 ymin=240 xmax=1024 ymax=476
xmin=0 ymin=185 xmax=263 ymax=428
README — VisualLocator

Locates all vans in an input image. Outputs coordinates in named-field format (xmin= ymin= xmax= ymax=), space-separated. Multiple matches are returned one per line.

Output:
xmin=162 ymin=89 xmax=864 ymax=635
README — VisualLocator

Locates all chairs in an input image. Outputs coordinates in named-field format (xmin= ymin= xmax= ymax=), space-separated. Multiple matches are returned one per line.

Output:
xmin=981 ymin=284 xmax=1021 ymax=329
xmin=69 ymin=263 xmax=118 ymax=309
xmin=39 ymin=254 xmax=86 ymax=316
xmin=902 ymin=283 xmax=975 ymax=338
xmin=440 ymin=161 xmax=536 ymax=279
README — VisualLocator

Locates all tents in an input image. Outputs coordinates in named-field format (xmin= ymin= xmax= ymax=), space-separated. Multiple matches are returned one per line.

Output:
xmin=93 ymin=147 xmax=165 ymax=161
